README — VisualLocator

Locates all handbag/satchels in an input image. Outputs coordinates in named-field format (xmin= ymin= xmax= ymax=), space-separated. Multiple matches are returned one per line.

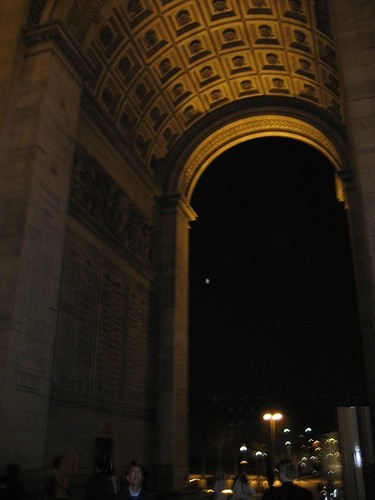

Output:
xmin=65 ymin=489 xmax=73 ymax=496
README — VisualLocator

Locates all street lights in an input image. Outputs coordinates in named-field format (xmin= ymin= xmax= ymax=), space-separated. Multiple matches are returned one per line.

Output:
xmin=263 ymin=412 xmax=283 ymax=483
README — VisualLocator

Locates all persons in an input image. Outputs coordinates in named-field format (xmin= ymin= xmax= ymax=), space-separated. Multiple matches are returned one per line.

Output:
xmin=117 ymin=461 xmax=149 ymax=500
xmin=261 ymin=458 xmax=316 ymax=500
